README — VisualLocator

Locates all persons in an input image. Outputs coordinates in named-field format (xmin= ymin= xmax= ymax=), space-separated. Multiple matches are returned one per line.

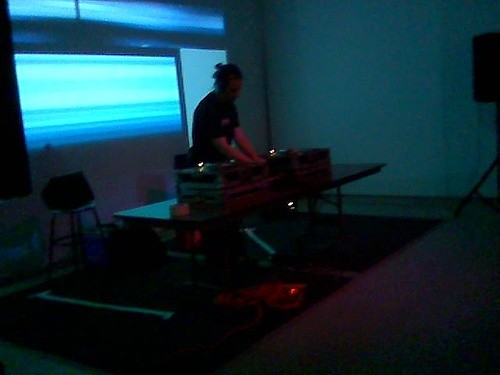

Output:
xmin=188 ymin=63 xmax=268 ymax=165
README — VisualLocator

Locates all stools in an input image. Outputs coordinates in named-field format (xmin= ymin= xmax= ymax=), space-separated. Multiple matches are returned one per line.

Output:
xmin=46 ymin=200 xmax=106 ymax=276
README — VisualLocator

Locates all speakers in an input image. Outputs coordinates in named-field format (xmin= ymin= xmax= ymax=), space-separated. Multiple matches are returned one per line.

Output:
xmin=472 ymin=32 xmax=500 ymax=103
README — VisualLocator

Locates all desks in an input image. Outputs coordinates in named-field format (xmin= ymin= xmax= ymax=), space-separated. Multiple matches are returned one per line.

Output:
xmin=112 ymin=160 xmax=385 ymax=304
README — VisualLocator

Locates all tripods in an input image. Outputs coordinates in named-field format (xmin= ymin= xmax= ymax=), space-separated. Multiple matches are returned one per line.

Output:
xmin=455 ymin=103 xmax=500 ymax=217
